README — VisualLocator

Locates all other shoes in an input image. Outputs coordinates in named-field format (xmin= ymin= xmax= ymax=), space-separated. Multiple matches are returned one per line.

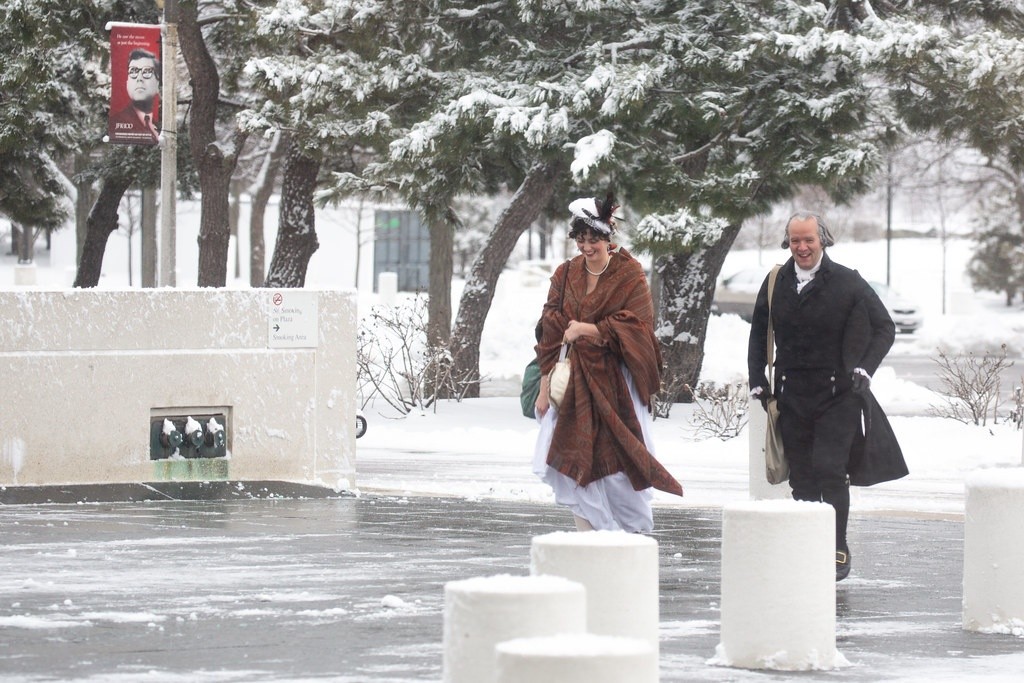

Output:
xmin=836 ymin=548 xmax=851 ymax=581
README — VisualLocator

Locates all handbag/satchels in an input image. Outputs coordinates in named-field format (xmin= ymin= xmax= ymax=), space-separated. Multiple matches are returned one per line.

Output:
xmin=847 ymin=389 xmax=910 ymax=486
xmin=520 ymin=360 xmax=549 ymax=418
xmin=766 ymin=394 xmax=790 ymax=484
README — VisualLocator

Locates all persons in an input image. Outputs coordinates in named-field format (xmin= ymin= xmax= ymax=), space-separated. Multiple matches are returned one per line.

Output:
xmin=532 ymin=212 xmax=683 ymax=535
xmin=746 ymin=211 xmax=909 ymax=581
xmin=111 ymin=48 xmax=162 ymax=144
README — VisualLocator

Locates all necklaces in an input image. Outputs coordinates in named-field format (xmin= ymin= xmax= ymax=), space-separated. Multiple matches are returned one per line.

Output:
xmin=585 ymin=255 xmax=612 ymax=276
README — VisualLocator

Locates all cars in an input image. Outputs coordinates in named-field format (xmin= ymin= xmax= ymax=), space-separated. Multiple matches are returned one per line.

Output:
xmin=710 ymin=264 xmax=921 ymax=335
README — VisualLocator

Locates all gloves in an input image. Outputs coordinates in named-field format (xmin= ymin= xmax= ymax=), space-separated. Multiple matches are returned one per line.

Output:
xmin=851 ymin=373 xmax=869 ymax=394
xmin=759 ymin=386 xmax=771 ymax=412
